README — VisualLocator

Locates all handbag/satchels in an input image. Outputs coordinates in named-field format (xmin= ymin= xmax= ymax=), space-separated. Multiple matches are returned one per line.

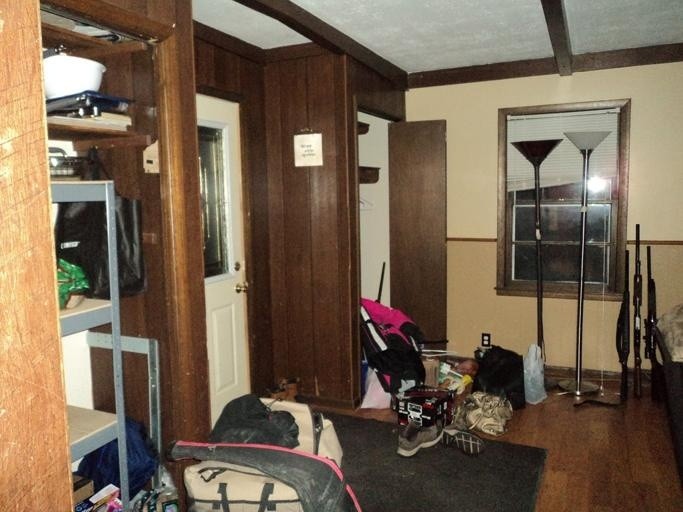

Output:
xmin=471 ymin=346 xmax=525 ymax=408
xmin=182 ymin=397 xmax=344 ymax=511
xmin=55 ymin=149 xmax=146 ymax=297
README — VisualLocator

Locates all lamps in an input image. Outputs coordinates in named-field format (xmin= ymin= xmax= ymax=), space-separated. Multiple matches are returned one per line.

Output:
xmin=510 ymin=140 xmax=561 ymax=391
xmin=558 ymin=131 xmax=612 ymax=397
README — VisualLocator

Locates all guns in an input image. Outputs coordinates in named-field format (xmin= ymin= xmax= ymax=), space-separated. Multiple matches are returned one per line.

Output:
xmin=616 ymin=223 xmax=664 ymax=403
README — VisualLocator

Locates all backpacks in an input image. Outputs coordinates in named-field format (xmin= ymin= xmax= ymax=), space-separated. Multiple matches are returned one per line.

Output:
xmin=360 ymin=297 xmax=426 ymax=392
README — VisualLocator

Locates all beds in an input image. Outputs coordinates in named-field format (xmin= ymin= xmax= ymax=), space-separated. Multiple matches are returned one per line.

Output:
xmin=650 ymin=304 xmax=682 ymax=485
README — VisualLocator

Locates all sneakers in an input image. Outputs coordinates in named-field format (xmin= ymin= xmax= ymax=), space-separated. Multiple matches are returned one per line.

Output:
xmin=467 ymin=390 xmax=514 ymax=436
xmin=397 ymin=422 xmax=485 ymax=457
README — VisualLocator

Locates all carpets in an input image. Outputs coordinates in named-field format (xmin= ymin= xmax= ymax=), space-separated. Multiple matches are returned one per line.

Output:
xmin=318 ymin=408 xmax=549 ymax=511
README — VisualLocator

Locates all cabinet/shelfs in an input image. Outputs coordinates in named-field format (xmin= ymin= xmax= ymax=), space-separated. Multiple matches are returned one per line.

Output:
xmin=264 ymin=55 xmax=447 ymax=408
xmin=41 ymin=19 xmax=154 ymax=151
xmin=52 ymin=182 xmax=129 ymax=512
